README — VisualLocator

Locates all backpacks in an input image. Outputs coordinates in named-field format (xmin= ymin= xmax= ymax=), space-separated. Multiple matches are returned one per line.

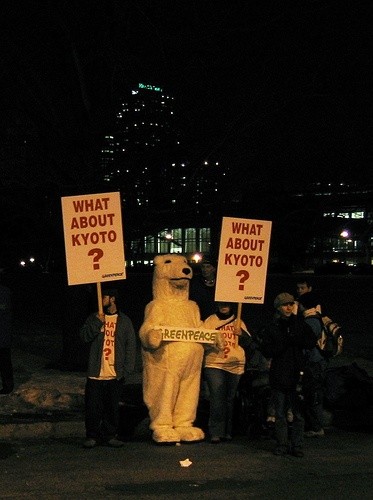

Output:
xmin=305 ymin=312 xmax=344 ymax=357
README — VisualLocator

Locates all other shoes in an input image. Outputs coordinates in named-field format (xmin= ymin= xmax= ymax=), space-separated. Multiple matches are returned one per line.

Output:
xmin=304 ymin=428 xmax=324 ymax=438
xmin=0 ymin=384 xmax=13 ymax=394
xmin=104 ymin=439 xmax=124 ymax=447
xmin=210 ymin=436 xmax=232 ymax=443
xmin=293 ymin=452 xmax=304 ymax=457
xmin=84 ymin=439 xmax=96 ymax=448
xmin=266 ymin=417 xmax=275 ymax=423
xmin=274 ymin=446 xmax=289 ymax=456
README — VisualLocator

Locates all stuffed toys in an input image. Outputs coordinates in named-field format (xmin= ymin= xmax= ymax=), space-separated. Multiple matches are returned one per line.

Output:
xmin=140 ymin=254 xmax=226 ymax=442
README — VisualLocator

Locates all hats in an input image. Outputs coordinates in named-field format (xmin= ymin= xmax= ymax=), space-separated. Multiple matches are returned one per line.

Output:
xmin=274 ymin=292 xmax=295 ymax=304
xmin=296 ymin=292 xmax=317 ymax=306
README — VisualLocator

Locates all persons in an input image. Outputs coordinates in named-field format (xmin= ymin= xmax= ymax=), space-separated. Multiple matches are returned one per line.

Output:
xmin=0 ymin=272 xmax=14 ymax=394
xmin=204 ymin=302 xmax=251 ymax=443
xmin=260 ymin=278 xmax=343 ymax=457
xmin=81 ymin=286 xmax=137 ymax=447
